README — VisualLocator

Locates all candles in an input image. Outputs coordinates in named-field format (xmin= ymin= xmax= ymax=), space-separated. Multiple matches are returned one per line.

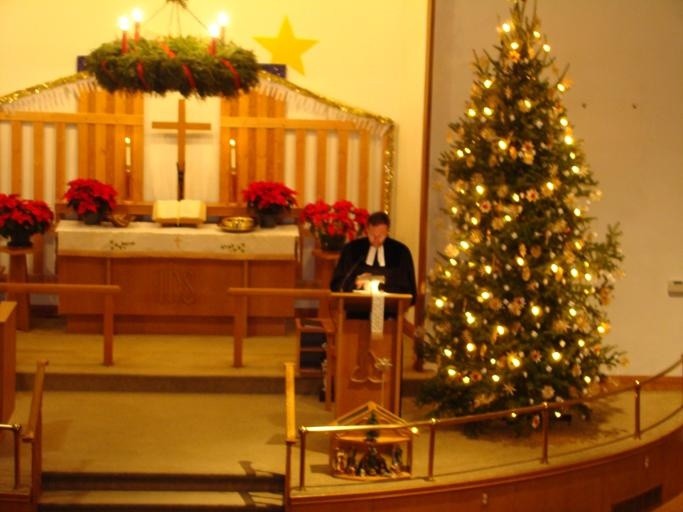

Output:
xmin=216 ymin=14 xmax=228 ymax=50
xmin=130 ymin=6 xmax=144 ymax=43
xmin=118 ymin=14 xmax=131 ymax=57
xmin=205 ymin=26 xmax=217 ymax=58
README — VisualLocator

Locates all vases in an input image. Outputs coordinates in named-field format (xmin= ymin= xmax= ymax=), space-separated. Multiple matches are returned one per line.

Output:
xmin=322 ymin=233 xmax=345 ymax=251
xmin=257 ymin=207 xmax=278 ymax=231
xmin=6 ymin=226 xmax=34 ymax=253
xmin=83 ymin=205 xmax=104 ymax=224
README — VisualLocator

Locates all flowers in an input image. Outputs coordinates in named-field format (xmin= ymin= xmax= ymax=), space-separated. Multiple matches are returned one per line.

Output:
xmin=0 ymin=190 xmax=53 ymax=235
xmin=239 ymin=180 xmax=299 ymax=214
xmin=60 ymin=178 xmax=120 ymax=213
xmin=299 ymin=195 xmax=372 ymax=241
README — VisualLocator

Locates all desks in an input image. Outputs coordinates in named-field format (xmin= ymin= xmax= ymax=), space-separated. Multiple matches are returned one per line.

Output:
xmin=53 ymin=218 xmax=300 ymax=337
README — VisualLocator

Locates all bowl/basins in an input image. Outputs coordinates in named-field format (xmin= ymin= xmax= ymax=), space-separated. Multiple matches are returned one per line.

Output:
xmin=216 ymin=215 xmax=257 ymax=233
xmin=106 ymin=214 xmax=134 ymax=229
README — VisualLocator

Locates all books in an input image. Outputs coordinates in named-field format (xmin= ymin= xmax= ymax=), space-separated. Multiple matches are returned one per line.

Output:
xmin=152 ymin=199 xmax=207 ymax=226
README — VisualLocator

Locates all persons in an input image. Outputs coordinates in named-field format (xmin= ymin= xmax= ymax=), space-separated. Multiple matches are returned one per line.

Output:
xmin=330 ymin=211 xmax=416 ymax=320
